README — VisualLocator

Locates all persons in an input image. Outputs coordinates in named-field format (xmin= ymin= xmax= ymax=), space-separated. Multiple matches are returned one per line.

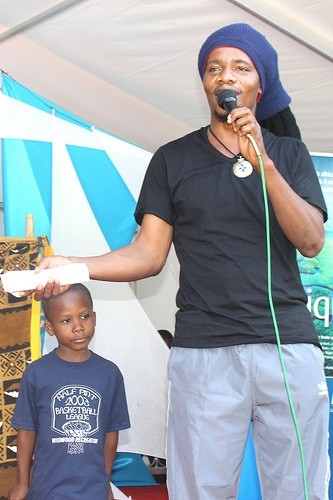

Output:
xmin=19 ymin=22 xmax=333 ymax=500
xmin=8 ymin=283 xmax=130 ymax=499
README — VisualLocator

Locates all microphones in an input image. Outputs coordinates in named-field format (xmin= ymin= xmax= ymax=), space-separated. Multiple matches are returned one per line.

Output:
xmin=217 ymin=89 xmax=251 ymax=137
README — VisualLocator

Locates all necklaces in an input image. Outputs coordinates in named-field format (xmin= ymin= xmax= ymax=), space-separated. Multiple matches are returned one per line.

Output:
xmin=209 ymin=125 xmax=254 ymax=179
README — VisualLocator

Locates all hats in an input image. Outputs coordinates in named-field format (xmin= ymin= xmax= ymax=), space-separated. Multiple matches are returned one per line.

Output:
xmin=197 ymin=23 xmax=291 ymax=122
